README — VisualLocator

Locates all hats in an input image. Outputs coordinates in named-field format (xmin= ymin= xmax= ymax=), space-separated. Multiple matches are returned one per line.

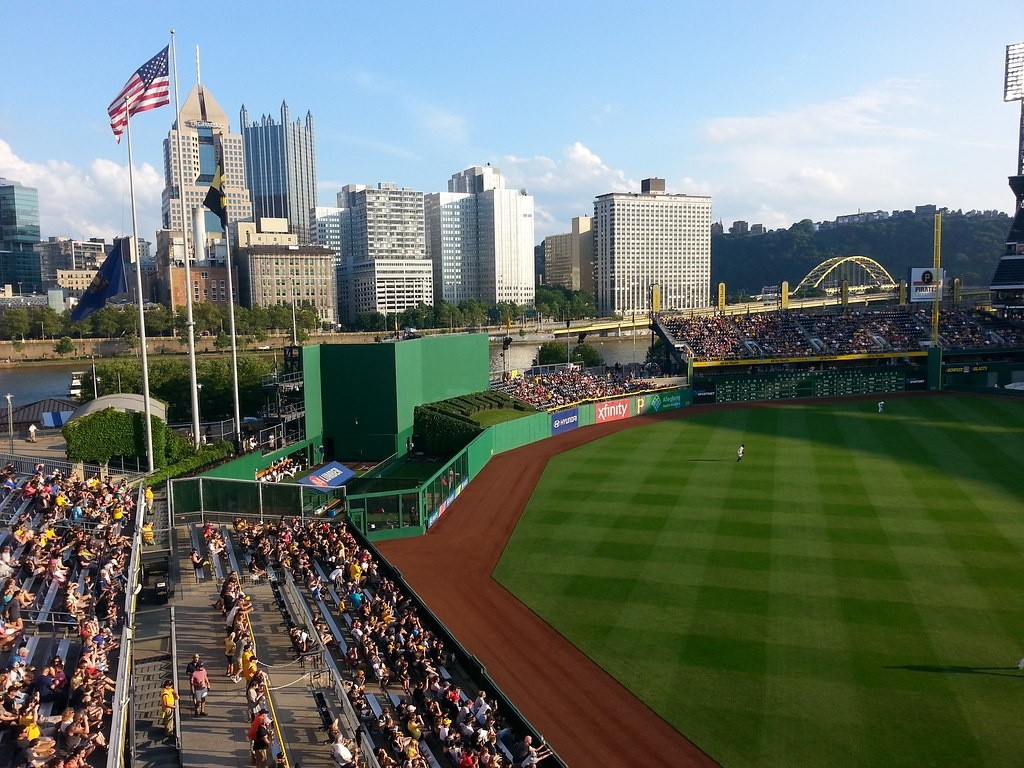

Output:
xmin=408 ymin=705 xmax=416 ymax=711
xmin=444 ymin=682 xmax=451 ymax=686
xmin=250 ymin=656 xmax=258 ymax=661
xmin=400 ymin=699 xmax=410 ymax=704
xmin=194 ymin=661 xmax=204 ymax=669
xmin=8 ymin=686 xmax=19 ymax=694
xmin=262 ymin=717 xmax=272 ymax=724
xmin=465 ymin=700 xmax=474 ymax=705
xmin=258 ymin=708 xmax=270 ymax=714
xmin=193 ymin=654 xmax=199 ymax=659
xmin=443 ymin=718 xmax=452 ymax=724
xmin=242 ymin=633 xmax=249 ymax=637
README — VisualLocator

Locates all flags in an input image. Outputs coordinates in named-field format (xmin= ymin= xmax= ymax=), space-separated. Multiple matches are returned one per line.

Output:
xmin=70 ymin=240 xmax=128 ymax=324
xmin=203 ymin=142 xmax=228 ymax=230
xmin=107 ymin=45 xmax=169 ymax=145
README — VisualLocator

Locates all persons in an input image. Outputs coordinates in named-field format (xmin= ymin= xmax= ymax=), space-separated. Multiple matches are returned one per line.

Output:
xmin=0 ymin=457 xmax=138 ymax=768
xmin=442 ymin=467 xmax=454 ymax=492
xmin=660 ymin=309 xmax=1023 ymax=362
xmin=737 ymin=444 xmax=745 ymax=462
xmin=259 ymin=456 xmax=302 ymax=484
xmin=186 ymin=654 xmax=211 ymax=716
xmin=29 ymin=421 xmax=37 ymax=443
xmin=878 ymin=400 xmax=886 ymax=413
xmin=204 ymin=425 xmax=212 ymax=444
xmin=191 ymin=547 xmax=210 ymax=569
xmin=141 ymin=520 xmax=156 ymax=547
xmin=160 ymin=680 xmax=181 ymax=742
xmin=498 ymin=362 xmax=688 ymax=412
xmin=204 ymin=515 xmax=563 ymax=768
xmin=409 ymin=507 xmax=417 ymax=527
xmin=268 ymin=432 xmax=274 ymax=451
xmin=238 ymin=430 xmax=258 ymax=455
xmin=281 ymin=436 xmax=286 ymax=447
xmin=144 ymin=485 xmax=156 ymax=515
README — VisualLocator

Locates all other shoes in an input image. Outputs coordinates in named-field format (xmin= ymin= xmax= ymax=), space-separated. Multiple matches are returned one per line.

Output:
xmin=225 ymin=673 xmax=231 ymax=677
xmin=195 ymin=710 xmax=200 ymax=718
xmin=212 ymin=604 xmax=218 ymax=609
xmin=236 ymin=676 xmax=242 ymax=682
xmin=201 ymin=712 xmax=208 ymax=716
xmin=231 ymin=676 xmax=237 ymax=683
